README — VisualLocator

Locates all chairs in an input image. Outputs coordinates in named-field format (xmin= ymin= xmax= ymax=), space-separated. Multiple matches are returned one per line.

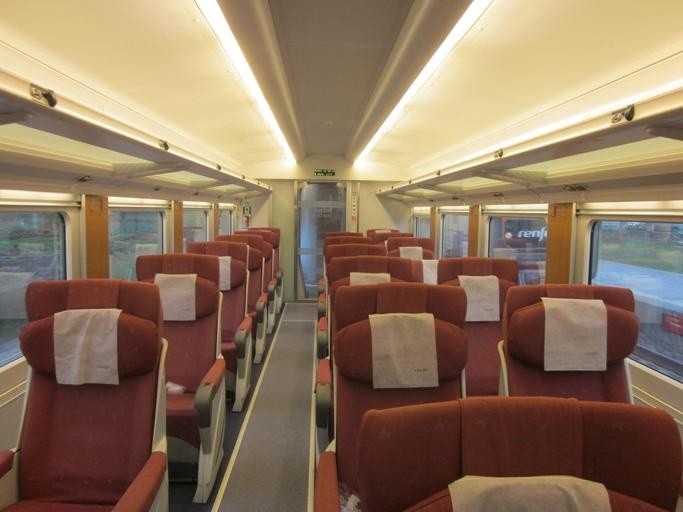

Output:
xmin=135 ymin=253 xmax=227 ymax=504
xmin=346 ymin=398 xmax=681 ymax=511
xmin=314 ymin=283 xmax=468 ymax=512
xmin=187 ymin=227 xmax=283 ymax=412
xmin=497 ymin=283 xmax=639 ymax=404
xmin=0 ymin=279 xmax=168 ymax=512
